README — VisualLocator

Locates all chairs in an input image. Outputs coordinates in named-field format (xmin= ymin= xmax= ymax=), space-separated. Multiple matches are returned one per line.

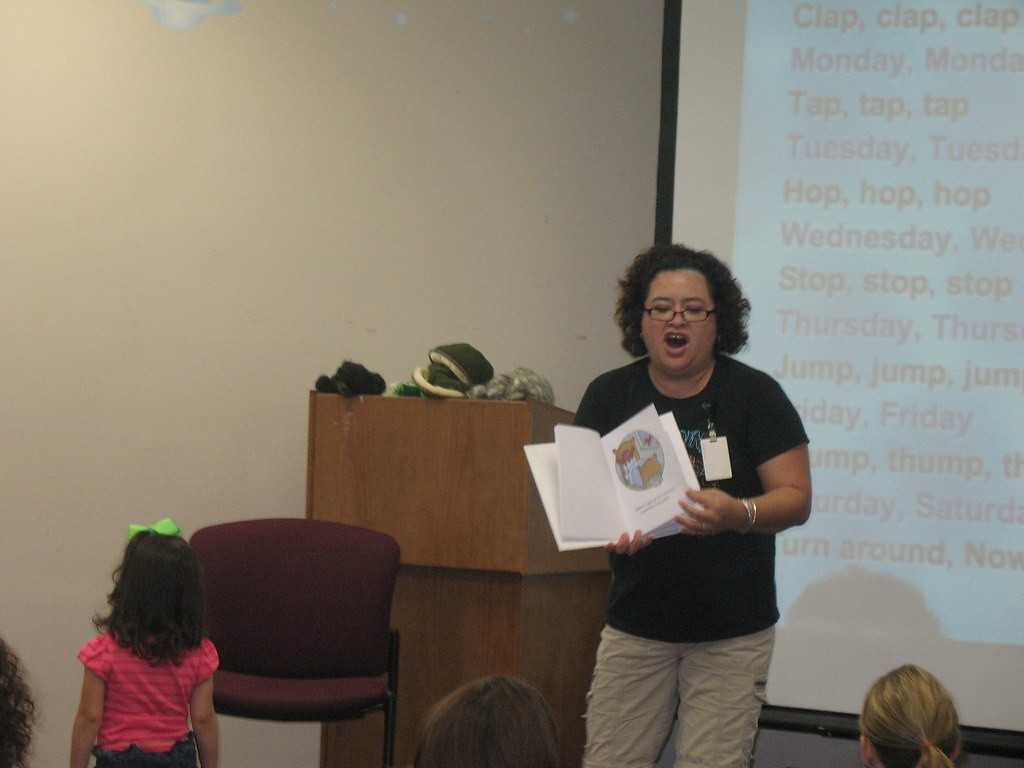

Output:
xmin=189 ymin=518 xmax=402 ymax=768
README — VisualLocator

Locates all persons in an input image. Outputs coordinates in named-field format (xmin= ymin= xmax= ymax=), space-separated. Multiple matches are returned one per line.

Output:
xmin=70 ymin=518 xmax=220 ymax=768
xmin=409 ymin=674 xmax=565 ymax=768
xmin=571 ymin=242 xmax=812 ymax=768
xmin=0 ymin=641 xmax=36 ymax=768
xmin=860 ymin=664 xmax=961 ymax=768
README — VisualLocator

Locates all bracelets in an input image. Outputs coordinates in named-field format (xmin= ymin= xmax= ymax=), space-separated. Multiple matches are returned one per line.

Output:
xmin=741 ymin=498 xmax=756 ymax=534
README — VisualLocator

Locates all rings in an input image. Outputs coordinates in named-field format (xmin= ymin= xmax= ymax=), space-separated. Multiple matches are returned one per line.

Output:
xmin=701 ymin=522 xmax=705 ymax=532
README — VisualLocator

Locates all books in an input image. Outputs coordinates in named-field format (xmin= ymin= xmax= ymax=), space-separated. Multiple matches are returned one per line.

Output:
xmin=524 ymin=402 xmax=704 ymax=552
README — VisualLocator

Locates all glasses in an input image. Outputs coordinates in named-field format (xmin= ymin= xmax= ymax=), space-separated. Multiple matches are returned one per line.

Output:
xmin=638 ymin=304 xmax=717 ymax=322
